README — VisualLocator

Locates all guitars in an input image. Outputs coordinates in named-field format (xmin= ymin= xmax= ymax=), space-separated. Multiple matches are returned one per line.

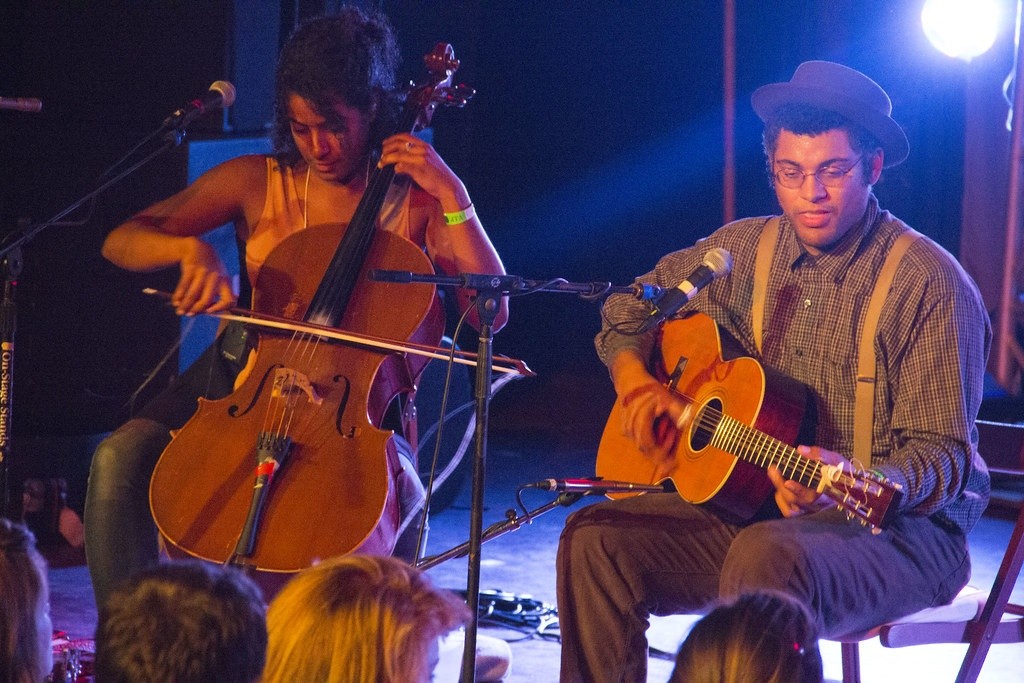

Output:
xmin=594 ymin=310 xmax=902 ymax=532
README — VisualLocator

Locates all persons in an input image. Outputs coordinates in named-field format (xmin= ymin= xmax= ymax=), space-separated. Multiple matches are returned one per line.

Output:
xmin=556 ymin=61 xmax=994 ymax=683
xmin=0 ymin=522 xmax=511 ymax=683
xmin=82 ymin=3 xmax=509 ymax=644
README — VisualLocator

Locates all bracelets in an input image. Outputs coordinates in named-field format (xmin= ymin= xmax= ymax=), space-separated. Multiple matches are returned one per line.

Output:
xmin=443 ymin=203 xmax=475 ymax=225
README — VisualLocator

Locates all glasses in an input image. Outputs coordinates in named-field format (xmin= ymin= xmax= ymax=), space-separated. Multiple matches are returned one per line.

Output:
xmin=772 ymin=153 xmax=867 ymax=189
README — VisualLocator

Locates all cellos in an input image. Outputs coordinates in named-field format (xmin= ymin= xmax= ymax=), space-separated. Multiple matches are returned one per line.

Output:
xmin=147 ymin=38 xmax=477 ymax=603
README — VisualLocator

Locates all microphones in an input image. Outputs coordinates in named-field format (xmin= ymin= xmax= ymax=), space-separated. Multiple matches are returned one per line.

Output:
xmin=635 ymin=246 xmax=732 ymax=333
xmin=537 ymin=478 xmax=665 ymax=493
xmin=165 ymin=81 xmax=236 ymax=129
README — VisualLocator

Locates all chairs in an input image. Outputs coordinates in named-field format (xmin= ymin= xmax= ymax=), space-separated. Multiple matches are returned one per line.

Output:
xmin=840 ymin=421 xmax=1024 ymax=683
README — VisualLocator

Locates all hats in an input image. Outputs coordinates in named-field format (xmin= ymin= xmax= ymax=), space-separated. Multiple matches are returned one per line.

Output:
xmin=750 ymin=60 xmax=910 ymax=168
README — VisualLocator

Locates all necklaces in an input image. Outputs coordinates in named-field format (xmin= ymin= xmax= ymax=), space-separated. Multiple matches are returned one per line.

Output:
xmin=305 ymin=159 xmax=369 ymax=228
